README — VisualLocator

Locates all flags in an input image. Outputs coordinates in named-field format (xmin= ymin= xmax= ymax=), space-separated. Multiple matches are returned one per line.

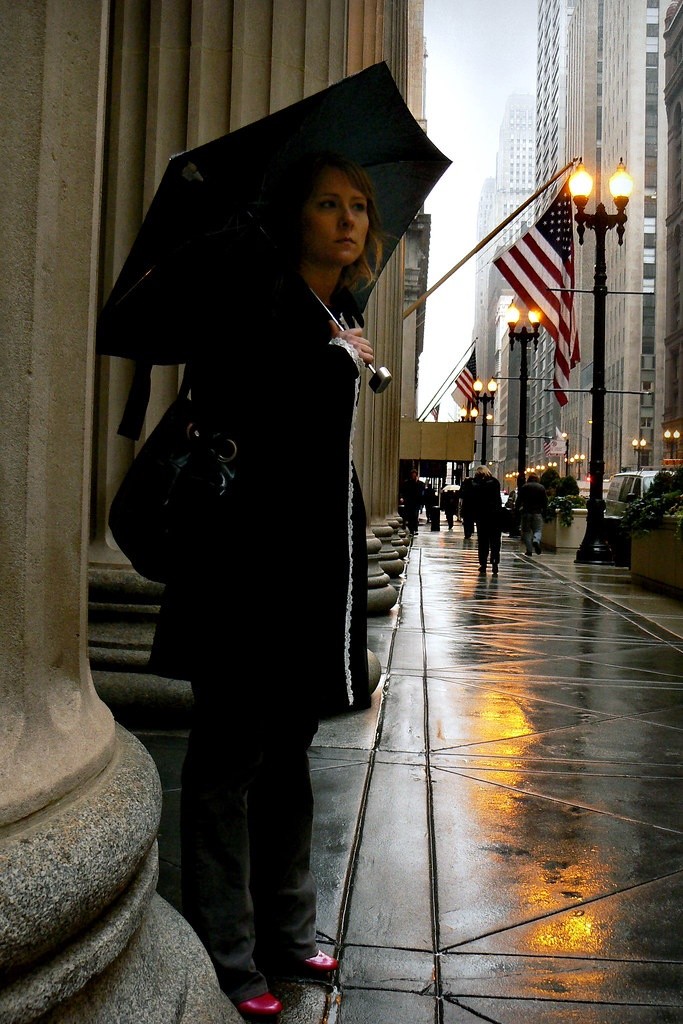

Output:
xmin=543 ymin=438 xmax=551 ymax=455
xmin=494 ymin=181 xmax=581 ymax=407
xmin=431 ymin=405 xmax=439 ymax=422
xmin=455 ymin=348 xmax=479 ymax=412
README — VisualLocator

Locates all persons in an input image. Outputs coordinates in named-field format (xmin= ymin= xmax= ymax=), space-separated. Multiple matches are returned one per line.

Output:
xmin=458 ymin=477 xmax=474 ymax=538
xmin=398 ymin=468 xmax=458 ymax=534
xmin=514 ymin=471 xmax=549 ymax=556
xmin=469 ymin=465 xmax=496 ymax=577
xmin=142 ymin=147 xmax=383 ymax=1014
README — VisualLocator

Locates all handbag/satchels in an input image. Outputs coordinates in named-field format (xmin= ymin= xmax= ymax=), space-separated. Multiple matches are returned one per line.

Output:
xmin=110 ymin=293 xmax=262 ymax=585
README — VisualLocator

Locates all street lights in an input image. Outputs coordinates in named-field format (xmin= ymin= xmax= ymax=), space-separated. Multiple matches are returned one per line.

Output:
xmin=562 ymin=432 xmax=590 ymax=481
xmin=589 ymin=419 xmax=623 ymax=473
xmin=504 ymin=299 xmax=543 ymax=539
xmin=664 ymin=429 xmax=680 ymax=460
xmin=505 ymin=471 xmax=520 ymax=495
xmin=473 ymin=375 xmax=498 ymax=465
xmin=631 ymin=438 xmax=647 ymax=472
xmin=488 ymin=460 xmax=505 ymax=491
xmin=570 ymin=156 xmax=635 ymax=566
xmin=458 ymin=406 xmax=479 ymax=478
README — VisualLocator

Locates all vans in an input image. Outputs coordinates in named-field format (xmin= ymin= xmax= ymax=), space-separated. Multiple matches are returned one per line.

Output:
xmin=606 ymin=472 xmax=676 ymax=519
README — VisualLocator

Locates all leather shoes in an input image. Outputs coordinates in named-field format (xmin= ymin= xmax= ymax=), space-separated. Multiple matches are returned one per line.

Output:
xmin=305 ymin=953 xmax=340 ymax=971
xmin=239 ymin=989 xmax=282 ymax=1016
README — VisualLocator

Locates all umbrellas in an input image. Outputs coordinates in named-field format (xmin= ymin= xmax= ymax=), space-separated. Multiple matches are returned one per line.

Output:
xmin=95 ymin=58 xmax=453 ymax=392
xmin=443 ymin=485 xmax=460 ymax=490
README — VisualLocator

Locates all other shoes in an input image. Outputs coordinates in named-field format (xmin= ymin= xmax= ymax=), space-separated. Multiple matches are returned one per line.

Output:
xmin=533 ymin=542 xmax=541 ymax=555
xmin=479 ymin=565 xmax=487 ymax=573
xmin=526 ymin=551 xmax=533 ymax=556
xmin=492 ymin=560 xmax=499 ymax=575
